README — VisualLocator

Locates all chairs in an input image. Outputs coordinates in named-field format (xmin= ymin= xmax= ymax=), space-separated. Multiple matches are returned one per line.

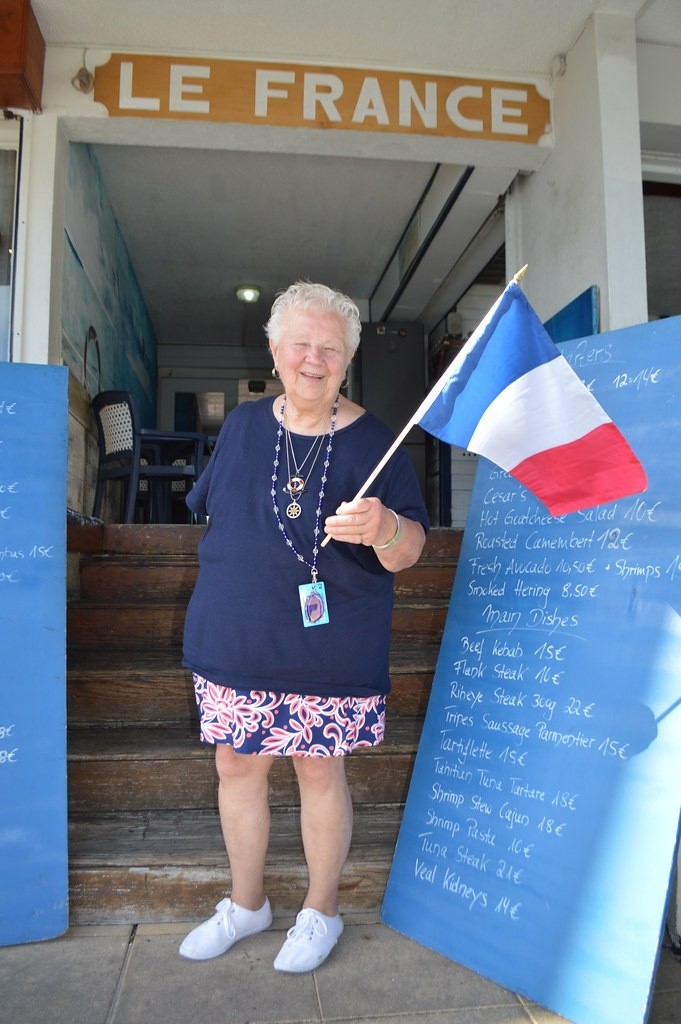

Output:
xmin=89 ymin=391 xmax=205 ymax=523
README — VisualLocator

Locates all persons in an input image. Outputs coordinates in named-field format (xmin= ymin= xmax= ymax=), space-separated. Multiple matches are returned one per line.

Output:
xmin=178 ymin=282 xmax=429 ymax=973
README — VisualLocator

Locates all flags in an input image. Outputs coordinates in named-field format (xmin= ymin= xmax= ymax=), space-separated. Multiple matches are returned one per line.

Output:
xmin=417 ymin=280 xmax=647 ymax=518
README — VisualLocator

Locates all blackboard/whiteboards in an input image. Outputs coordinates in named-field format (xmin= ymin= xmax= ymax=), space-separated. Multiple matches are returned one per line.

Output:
xmin=379 ymin=313 xmax=680 ymax=1024
xmin=0 ymin=362 xmax=70 ymax=947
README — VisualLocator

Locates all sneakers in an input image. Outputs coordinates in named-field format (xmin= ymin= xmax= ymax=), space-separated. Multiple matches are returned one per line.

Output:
xmin=274 ymin=907 xmax=344 ymax=972
xmin=179 ymin=895 xmax=273 ymax=960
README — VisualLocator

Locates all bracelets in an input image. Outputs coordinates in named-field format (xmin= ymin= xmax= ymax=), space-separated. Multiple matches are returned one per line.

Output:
xmin=372 ymin=509 xmax=401 ymax=550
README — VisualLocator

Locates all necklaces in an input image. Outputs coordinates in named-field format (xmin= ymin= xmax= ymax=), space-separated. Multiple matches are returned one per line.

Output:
xmin=271 ymin=394 xmax=340 ymax=627
